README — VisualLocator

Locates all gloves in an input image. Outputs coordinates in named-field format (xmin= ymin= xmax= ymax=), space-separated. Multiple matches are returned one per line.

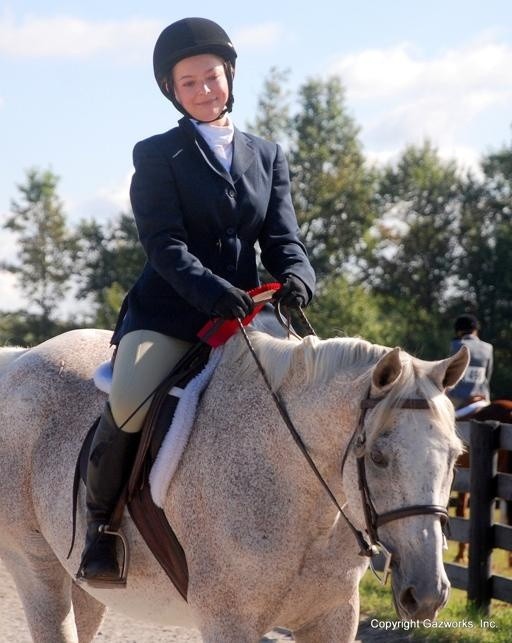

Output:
xmin=273 ymin=273 xmax=309 ymax=308
xmin=212 ymin=287 xmax=253 ymax=319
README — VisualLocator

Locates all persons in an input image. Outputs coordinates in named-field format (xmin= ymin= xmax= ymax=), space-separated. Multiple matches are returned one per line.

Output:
xmin=76 ymin=17 xmax=317 ymax=585
xmin=450 ymin=312 xmax=495 ymax=406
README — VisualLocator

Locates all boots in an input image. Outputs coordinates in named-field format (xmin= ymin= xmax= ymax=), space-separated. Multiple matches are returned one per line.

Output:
xmin=76 ymin=403 xmax=142 ymax=581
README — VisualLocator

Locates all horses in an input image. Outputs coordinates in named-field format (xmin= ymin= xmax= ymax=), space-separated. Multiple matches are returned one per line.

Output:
xmin=453 ymin=400 xmax=512 ymax=565
xmin=0 ymin=328 xmax=470 ymax=643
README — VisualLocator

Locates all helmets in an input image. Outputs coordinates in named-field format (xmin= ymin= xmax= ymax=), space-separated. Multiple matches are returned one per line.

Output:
xmin=153 ymin=17 xmax=237 ymax=81
